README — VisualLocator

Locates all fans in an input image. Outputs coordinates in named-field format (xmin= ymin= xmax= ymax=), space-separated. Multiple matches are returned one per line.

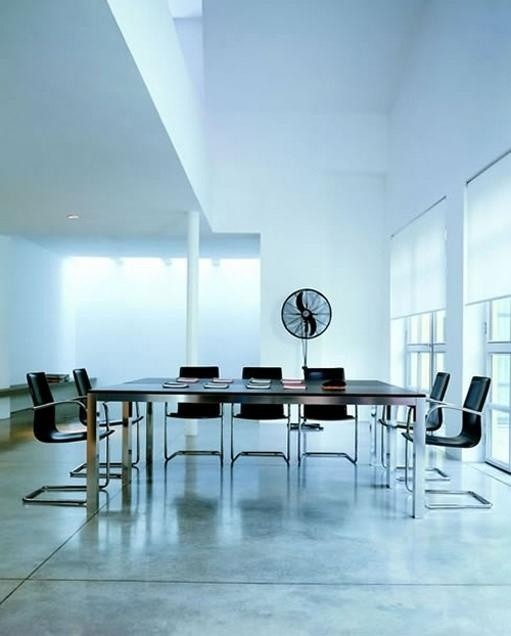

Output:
xmin=280 ymin=287 xmax=331 ymax=430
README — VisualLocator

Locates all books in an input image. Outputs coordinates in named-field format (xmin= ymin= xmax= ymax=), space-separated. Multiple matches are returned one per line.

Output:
xmin=45 ymin=373 xmax=69 ymax=383
xmin=321 ymin=384 xmax=346 ymax=390
xmin=176 ymin=376 xmax=199 ymax=383
xmin=246 ymin=383 xmax=270 ymax=389
xmin=161 ymin=380 xmax=188 ymax=388
xmin=247 ymin=376 xmax=272 ymax=384
xmin=281 ymin=378 xmax=302 ymax=385
xmin=203 ymin=381 xmax=228 ymax=389
xmin=282 ymin=383 xmax=307 ymax=390
xmin=212 ymin=377 xmax=233 ymax=383
